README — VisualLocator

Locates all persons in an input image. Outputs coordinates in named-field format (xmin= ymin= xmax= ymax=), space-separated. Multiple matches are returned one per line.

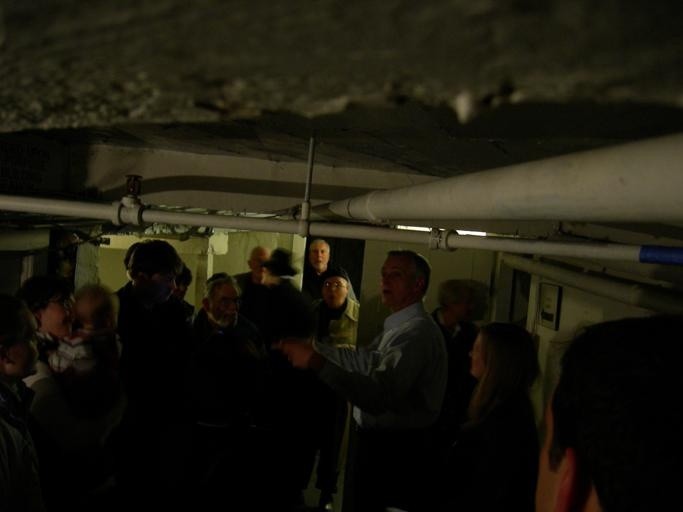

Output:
xmin=233 ymin=246 xmax=270 ymax=327
xmin=429 ymin=319 xmax=540 ymax=512
xmin=168 ymin=262 xmax=194 ymax=512
xmin=534 ymin=314 xmax=682 ymax=511
xmin=303 ymin=268 xmax=359 ymax=510
xmin=279 ymin=249 xmax=449 ymax=511
xmin=1 ymin=272 xmax=119 ymax=510
xmin=189 ymin=272 xmax=262 ymax=512
xmin=433 ymin=278 xmax=479 ymax=435
xmin=303 ymin=239 xmax=346 ymax=303
xmin=258 ymin=246 xmax=300 ymax=337
xmin=115 ymin=239 xmax=182 ymax=511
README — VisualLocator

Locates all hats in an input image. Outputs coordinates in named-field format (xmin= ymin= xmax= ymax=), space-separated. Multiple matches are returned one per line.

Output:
xmin=261 ymin=249 xmax=296 ymax=277
xmin=320 ymin=265 xmax=348 ymax=282
